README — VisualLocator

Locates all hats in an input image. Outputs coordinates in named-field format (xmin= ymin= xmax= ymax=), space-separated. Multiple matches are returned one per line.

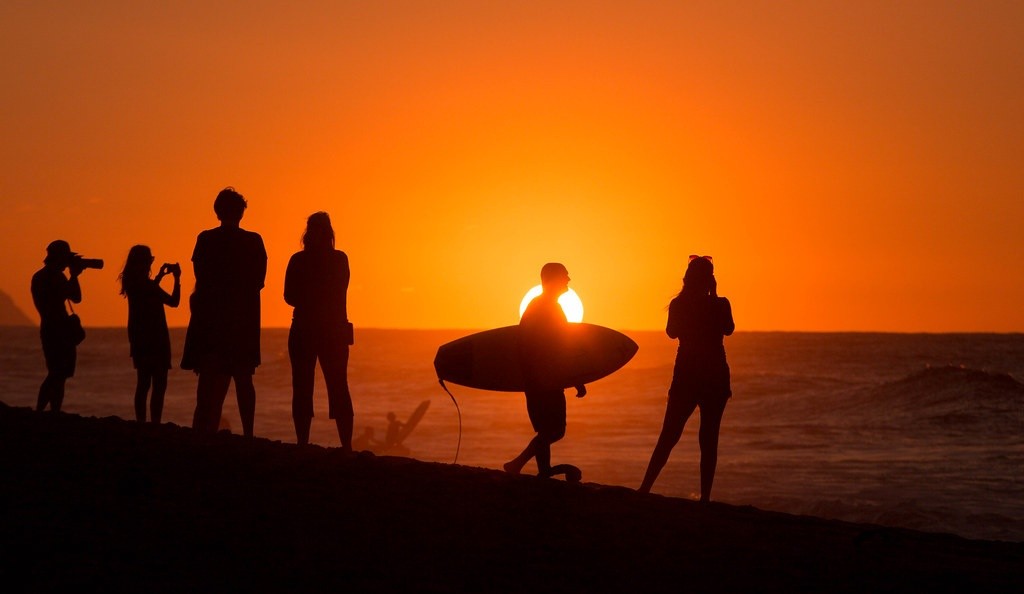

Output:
xmin=47 ymin=240 xmax=77 ymax=258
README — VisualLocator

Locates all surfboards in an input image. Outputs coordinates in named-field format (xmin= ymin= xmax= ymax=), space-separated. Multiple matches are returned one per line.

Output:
xmin=434 ymin=322 xmax=639 ymax=392
xmin=393 ymin=399 xmax=432 ymax=443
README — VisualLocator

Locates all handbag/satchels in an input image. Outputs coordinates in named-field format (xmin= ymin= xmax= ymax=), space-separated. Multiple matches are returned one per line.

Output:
xmin=67 ymin=314 xmax=88 ymax=346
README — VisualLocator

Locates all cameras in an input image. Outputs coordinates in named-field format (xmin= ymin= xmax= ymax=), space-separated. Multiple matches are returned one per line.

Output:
xmin=166 ymin=264 xmax=176 ymax=271
xmin=68 ymin=256 xmax=103 ymax=269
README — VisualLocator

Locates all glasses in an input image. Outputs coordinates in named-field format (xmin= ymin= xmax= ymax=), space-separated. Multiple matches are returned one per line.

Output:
xmin=150 ymin=256 xmax=155 ymax=264
xmin=688 ymin=254 xmax=714 ymax=265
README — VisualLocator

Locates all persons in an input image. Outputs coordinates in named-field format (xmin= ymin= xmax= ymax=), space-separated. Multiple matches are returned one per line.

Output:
xmin=503 ymin=263 xmax=586 ymax=482
xmin=117 ymin=244 xmax=182 ymax=424
xmin=637 ymin=255 xmax=735 ymax=501
xmin=180 ymin=186 xmax=267 ymax=436
xmin=284 ymin=211 xmax=355 ymax=449
xmin=31 ymin=240 xmax=86 ymax=411
